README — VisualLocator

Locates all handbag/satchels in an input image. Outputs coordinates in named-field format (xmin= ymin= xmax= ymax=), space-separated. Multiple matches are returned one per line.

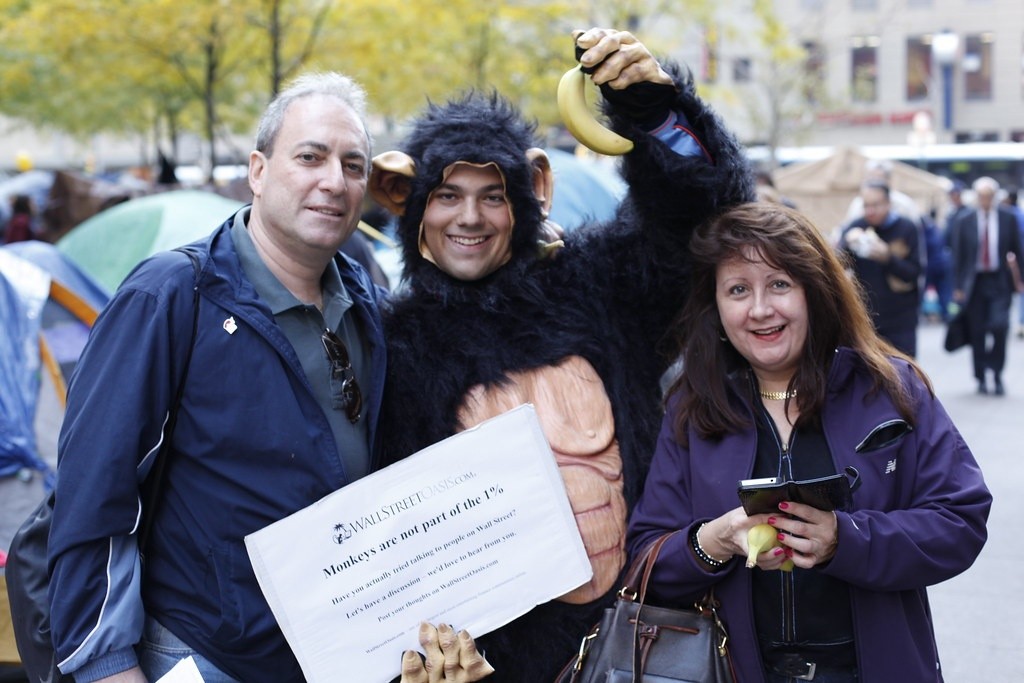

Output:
xmin=553 ymin=531 xmax=736 ymax=683
xmin=5 ymin=489 xmax=150 ymax=683
xmin=945 ymin=314 xmax=971 ymax=352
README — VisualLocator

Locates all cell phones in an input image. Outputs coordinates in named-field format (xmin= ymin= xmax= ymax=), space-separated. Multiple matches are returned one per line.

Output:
xmin=736 ymin=466 xmax=862 ymax=523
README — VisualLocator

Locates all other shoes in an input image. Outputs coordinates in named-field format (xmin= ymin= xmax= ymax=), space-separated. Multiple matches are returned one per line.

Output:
xmin=978 ymin=379 xmax=987 ymax=393
xmin=995 ymin=377 xmax=1003 ymax=394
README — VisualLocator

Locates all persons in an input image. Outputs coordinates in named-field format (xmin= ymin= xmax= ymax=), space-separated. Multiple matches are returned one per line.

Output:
xmin=926 ymin=175 xmax=1024 ymax=398
xmin=372 ymin=26 xmax=758 ymax=683
xmin=830 ymin=159 xmax=928 ymax=362
xmin=152 ymin=151 xmax=182 ymax=191
xmin=618 ymin=202 xmax=993 ymax=683
xmin=45 ymin=71 xmax=395 ymax=683
xmin=0 ymin=171 xmax=131 ymax=245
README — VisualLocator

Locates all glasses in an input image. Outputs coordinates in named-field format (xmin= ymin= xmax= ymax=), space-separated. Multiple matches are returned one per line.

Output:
xmin=321 ymin=327 xmax=362 ymax=424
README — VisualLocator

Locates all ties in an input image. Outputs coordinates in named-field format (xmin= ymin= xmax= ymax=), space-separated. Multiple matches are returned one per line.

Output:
xmin=983 ymin=222 xmax=989 ymax=271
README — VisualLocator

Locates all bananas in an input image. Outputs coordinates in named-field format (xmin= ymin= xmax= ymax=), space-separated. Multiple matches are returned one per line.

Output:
xmin=556 ymin=62 xmax=634 ymax=156
xmin=746 ymin=524 xmax=794 ymax=572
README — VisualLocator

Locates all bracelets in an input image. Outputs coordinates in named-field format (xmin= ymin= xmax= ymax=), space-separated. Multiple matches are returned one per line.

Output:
xmin=691 ymin=522 xmax=732 ymax=565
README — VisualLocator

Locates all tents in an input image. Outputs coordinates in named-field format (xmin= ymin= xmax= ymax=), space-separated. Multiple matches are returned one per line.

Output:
xmin=379 ymin=140 xmax=627 ymax=271
xmin=769 ymin=144 xmax=957 ymax=254
xmin=0 ymin=240 xmax=116 ymax=490
xmin=53 ymin=190 xmax=250 ymax=296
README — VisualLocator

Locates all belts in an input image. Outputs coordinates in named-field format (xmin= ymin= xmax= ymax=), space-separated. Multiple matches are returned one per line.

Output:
xmin=760 ymin=649 xmax=857 ymax=681
